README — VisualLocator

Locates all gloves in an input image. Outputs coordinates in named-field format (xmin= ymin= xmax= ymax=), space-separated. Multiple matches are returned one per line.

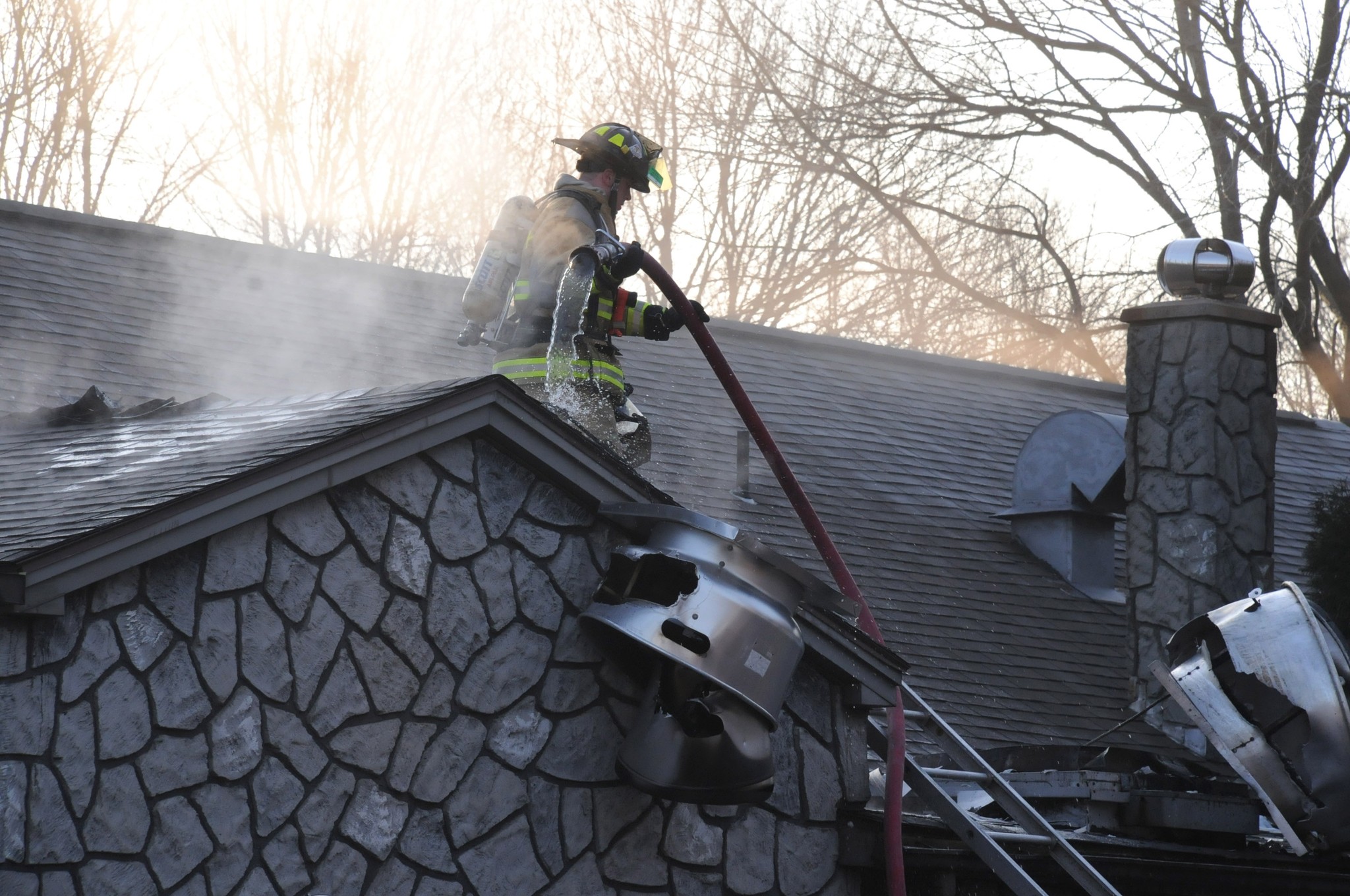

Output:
xmin=661 ymin=300 xmax=709 ymax=333
xmin=611 ymin=240 xmax=644 ymax=281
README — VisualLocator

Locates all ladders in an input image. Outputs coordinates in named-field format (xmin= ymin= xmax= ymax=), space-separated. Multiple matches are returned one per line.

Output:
xmin=863 ymin=679 xmax=1123 ymax=895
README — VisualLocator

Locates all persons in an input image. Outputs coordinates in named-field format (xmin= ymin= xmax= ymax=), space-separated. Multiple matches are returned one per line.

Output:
xmin=491 ymin=122 xmax=709 ymax=469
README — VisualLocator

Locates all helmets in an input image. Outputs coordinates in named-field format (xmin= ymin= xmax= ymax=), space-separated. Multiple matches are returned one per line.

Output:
xmin=550 ymin=125 xmax=656 ymax=194
